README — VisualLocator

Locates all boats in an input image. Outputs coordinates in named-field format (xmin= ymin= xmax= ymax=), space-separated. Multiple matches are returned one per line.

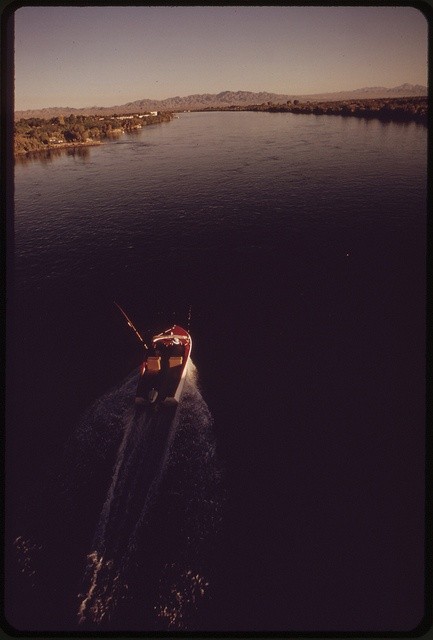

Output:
xmin=113 ymin=299 xmax=192 ymax=409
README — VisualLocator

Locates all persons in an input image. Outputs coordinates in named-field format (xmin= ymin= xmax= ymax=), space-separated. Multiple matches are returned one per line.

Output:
xmin=170 ymin=337 xmax=182 ymax=346
